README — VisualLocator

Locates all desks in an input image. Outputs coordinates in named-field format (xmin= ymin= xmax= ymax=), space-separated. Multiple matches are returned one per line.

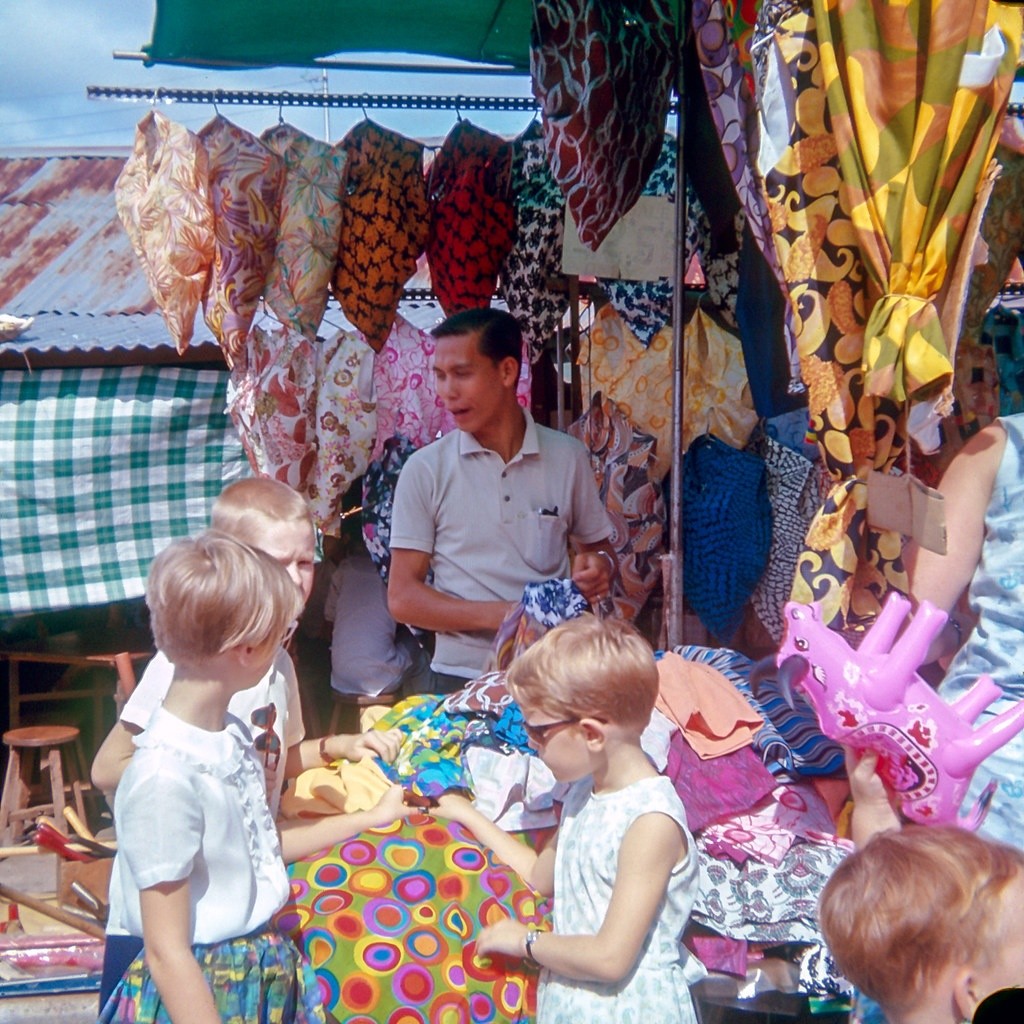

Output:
xmin=0 ymin=643 xmax=154 ymax=754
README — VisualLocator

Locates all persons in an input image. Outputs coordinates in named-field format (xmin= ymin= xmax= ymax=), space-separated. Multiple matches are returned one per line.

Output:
xmin=906 ymin=412 xmax=1024 ymax=853
xmin=429 ymin=611 xmax=709 ymax=1024
xmin=386 ymin=308 xmax=620 ymax=695
xmin=91 ymin=476 xmax=435 ymax=1024
xmin=817 ymin=743 xmax=1024 ymax=1024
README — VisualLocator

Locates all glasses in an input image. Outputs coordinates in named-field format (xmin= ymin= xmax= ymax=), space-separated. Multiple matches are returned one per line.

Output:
xmin=251 ymin=703 xmax=280 ymax=770
xmin=522 ymin=714 xmax=608 ymax=748
xmin=543 ymin=506 xmax=558 ymax=517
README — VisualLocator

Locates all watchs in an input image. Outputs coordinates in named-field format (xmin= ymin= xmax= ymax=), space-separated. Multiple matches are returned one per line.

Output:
xmin=526 ymin=929 xmax=544 ymax=969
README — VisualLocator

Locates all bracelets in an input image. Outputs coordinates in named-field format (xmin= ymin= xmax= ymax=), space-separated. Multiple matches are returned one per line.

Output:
xmin=598 ymin=550 xmax=615 ymax=575
xmin=949 ymin=617 xmax=962 ymax=653
xmin=319 ymin=734 xmax=336 ymax=763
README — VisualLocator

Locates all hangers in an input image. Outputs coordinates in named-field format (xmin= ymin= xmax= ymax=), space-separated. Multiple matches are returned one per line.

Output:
xmin=695 ymin=406 xmax=715 ymax=444
xmin=753 ymin=417 xmax=767 ymax=443
xmin=247 ymin=298 xmax=346 ymax=350
xmin=386 ymin=406 xmax=410 ymax=450
xmin=143 ymin=87 xmax=541 ymax=188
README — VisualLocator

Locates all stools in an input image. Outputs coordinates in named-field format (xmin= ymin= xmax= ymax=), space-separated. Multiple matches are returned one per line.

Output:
xmin=326 ymin=690 xmax=395 ymax=739
xmin=0 ymin=724 xmax=98 ymax=848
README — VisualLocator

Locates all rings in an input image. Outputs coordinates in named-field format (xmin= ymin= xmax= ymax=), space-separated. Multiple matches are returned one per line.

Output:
xmin=596 ymin=594 xmax=601 ymax=603
